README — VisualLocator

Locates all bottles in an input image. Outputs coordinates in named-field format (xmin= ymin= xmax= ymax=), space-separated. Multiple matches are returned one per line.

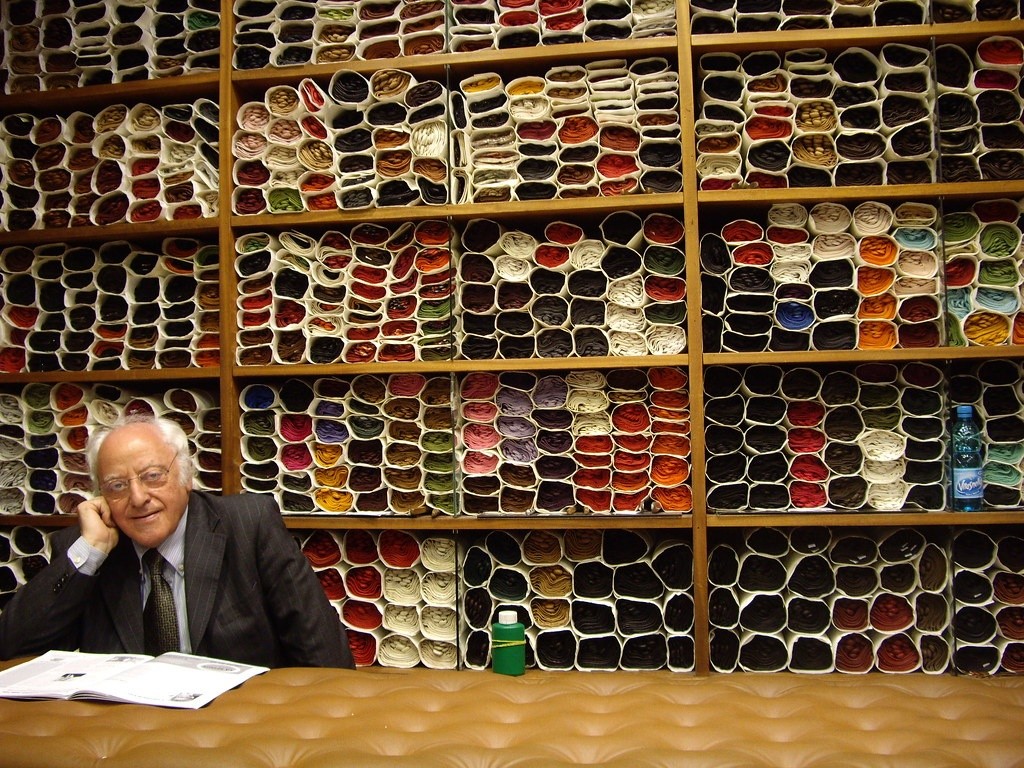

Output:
xmin=949 ymin=406 xmax=985 ymax=512
xmin=490 ymin=610 xmax=526 ymax=675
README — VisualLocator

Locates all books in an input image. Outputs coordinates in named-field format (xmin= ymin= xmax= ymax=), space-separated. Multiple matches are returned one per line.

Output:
xmin=0 ymin=650 xmax=270 ymax=711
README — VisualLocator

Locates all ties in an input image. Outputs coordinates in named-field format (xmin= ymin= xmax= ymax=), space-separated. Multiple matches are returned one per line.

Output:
xmin=144 ymin=548 xmax=180 ymax=653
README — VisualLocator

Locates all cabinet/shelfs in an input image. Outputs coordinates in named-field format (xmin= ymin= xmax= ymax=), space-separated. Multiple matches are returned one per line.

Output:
xmin=447 ymin=0 xmax=1024 ymax=682
xmin=0 ymin=0 xmax=453 ymax=668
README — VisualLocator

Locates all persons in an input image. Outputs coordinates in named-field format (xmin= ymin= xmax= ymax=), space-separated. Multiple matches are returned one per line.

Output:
xmin=1 ymin=415 xmax=357 ymax=670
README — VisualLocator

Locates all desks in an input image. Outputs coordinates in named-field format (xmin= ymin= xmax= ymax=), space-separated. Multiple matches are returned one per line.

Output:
xmin=0 ymin=657 xmax=1024 ymax=768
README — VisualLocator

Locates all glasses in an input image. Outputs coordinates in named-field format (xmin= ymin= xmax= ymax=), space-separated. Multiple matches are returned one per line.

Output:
xmin=98 ymin=450 xmax=179 ymax=503
